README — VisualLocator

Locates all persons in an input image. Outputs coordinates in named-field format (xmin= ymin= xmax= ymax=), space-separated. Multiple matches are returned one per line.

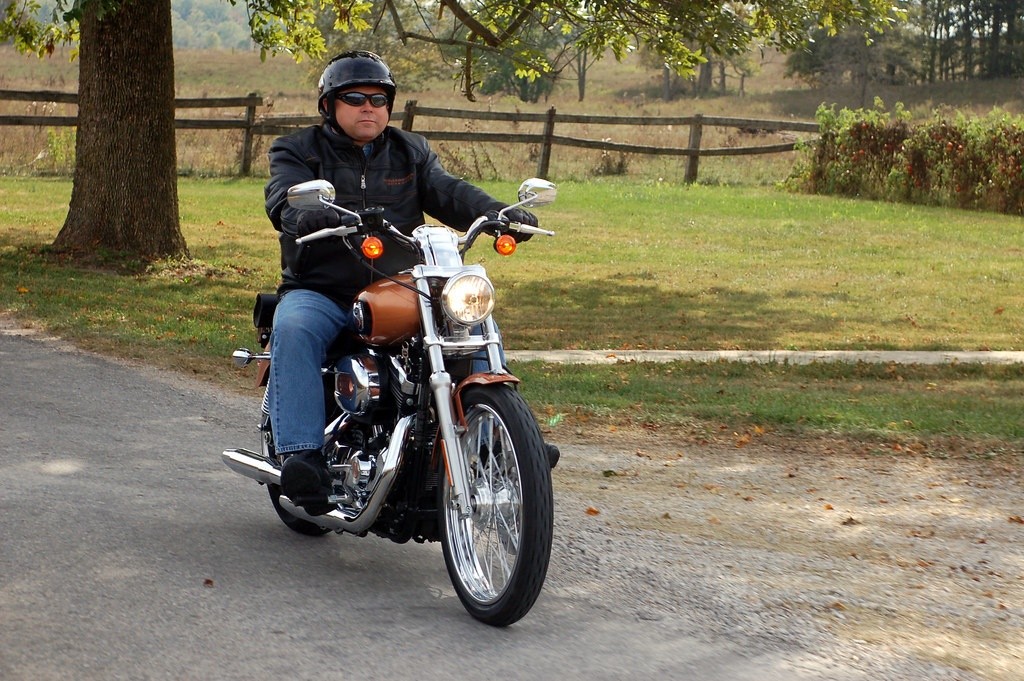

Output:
xmin=264 ymin=50 xmax=560 ymax=516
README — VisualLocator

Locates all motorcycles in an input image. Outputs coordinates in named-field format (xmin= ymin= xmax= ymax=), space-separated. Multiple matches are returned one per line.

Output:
xmin=221 ymin=176 xmax=561 ymax=626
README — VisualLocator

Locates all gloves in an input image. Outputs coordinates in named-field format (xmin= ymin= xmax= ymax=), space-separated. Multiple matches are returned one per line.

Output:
xmin=297 ymin=206 xmax=339 ymax=249
xmin=490 ymin=206 xmax=539 ymax=242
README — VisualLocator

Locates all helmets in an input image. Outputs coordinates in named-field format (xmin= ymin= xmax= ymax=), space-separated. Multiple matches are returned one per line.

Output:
xmin=318 ymin=50 xmax=397 ymax=113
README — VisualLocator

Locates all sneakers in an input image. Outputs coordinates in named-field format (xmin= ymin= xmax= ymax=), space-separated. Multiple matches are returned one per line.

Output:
xmin=281 ymin=449 xmax=329 ymax=517
xmin=473 ymin=437 xmax=561 ymax=479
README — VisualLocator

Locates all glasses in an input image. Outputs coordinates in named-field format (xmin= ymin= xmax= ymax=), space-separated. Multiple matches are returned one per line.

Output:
xmin=335 ymin=91 xmax=388 ymax=107
